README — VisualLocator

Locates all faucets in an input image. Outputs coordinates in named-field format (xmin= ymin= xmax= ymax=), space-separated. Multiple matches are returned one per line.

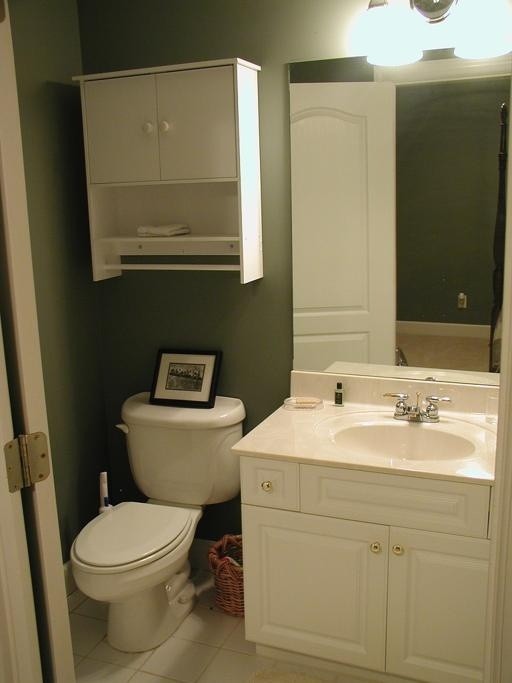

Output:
xmin=406 ymin=405 xmax=422 ymax=421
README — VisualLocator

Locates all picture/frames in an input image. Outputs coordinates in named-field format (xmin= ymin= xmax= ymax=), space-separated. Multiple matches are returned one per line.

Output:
xmin=147 ymin=345 xmax=223 ymax=409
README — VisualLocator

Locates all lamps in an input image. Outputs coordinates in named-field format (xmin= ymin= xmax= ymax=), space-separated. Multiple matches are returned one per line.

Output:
xmin=342 ymin=2 xmax=512 ymax=68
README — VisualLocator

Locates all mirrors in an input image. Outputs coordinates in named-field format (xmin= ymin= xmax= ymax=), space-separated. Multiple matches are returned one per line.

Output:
xmin=287 ymin=42 xmax=510 ymax=406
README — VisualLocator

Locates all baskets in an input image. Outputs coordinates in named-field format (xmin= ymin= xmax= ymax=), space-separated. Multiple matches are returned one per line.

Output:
xmin=206 ymin=532 xmax=245 ymax=618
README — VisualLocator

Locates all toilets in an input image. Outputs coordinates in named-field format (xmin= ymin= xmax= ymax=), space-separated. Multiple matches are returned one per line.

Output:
xmin=70 ymin=390 xmax=246 ymax=654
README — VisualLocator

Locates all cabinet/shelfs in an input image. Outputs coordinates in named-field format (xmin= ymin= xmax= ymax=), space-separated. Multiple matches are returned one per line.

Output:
xmin=232 ymin=448 xmax=511 ymax=678
xmin=70 ymin=56 xmax=266 ymax=285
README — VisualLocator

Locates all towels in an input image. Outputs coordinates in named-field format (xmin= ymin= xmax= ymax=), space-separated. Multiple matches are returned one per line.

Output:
xmin=134 ymin=222 xmax=194 ymax=238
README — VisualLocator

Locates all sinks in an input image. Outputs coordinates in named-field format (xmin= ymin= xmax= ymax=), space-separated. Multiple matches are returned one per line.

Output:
xmin=335 ymin=424 xmax=475 ymax=461
